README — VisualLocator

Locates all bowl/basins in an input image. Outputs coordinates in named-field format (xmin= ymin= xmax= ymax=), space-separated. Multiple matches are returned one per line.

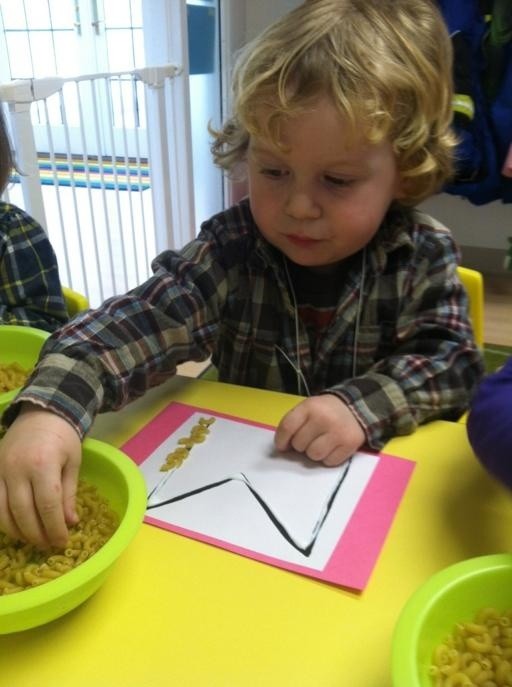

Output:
xmin=0 ymin=430 xmax=148 ymax=636
xmin=0 ymin=325 xmax=53 ymax=420
xmin=390 ymin=554 xmax=512 ymax=687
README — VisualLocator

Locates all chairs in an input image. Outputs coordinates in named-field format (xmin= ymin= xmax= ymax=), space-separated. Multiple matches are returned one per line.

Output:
xmin=457 ymin=266 xmax=484 ymax=350
xmin=60 ymin=287 xmax=89 ymax=319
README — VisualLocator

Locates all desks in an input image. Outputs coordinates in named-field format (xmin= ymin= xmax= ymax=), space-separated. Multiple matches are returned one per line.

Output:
xmin=1 ymin=374 xmax=512 ymax=687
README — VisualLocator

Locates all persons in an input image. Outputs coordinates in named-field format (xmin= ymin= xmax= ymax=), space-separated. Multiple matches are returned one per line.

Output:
xmin=469 ymin=353 xmax=511 ymax=484
xmin=0 ymin=1 xmax=482 ymax=553
xmin=0 ymin=103 xmax=70 ymax=334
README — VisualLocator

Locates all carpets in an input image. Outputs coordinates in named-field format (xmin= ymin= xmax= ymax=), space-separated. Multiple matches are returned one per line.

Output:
xmin=197 ymin=343 xmax=511 ymax=382
